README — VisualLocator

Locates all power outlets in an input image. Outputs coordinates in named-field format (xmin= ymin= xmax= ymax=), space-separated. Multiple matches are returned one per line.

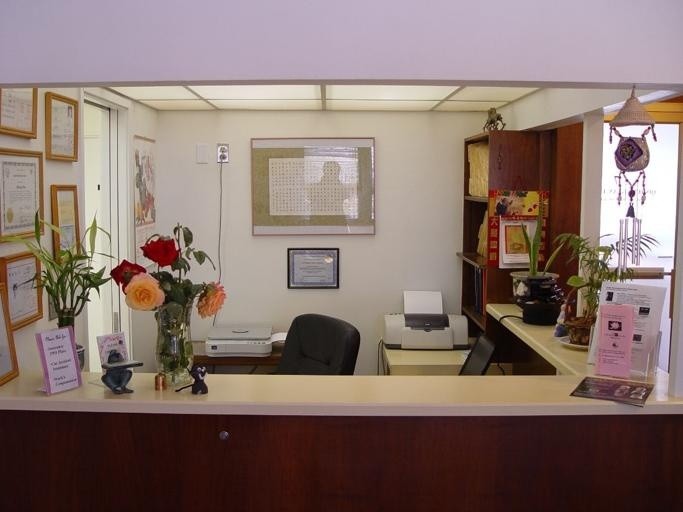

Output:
xmin=216 ymin=143 xmax=230 ymax=163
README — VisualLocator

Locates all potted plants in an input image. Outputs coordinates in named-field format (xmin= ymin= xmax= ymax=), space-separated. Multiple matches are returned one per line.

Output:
xmin=1 ymin=212 xmax=114 ymax=371
xmin=509 ymin=204 xmax=567 ymax=307
xmin=553 ymin=234 xmax=660 ymax=345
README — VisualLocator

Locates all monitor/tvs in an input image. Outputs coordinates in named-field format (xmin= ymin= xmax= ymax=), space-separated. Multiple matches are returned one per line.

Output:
xmin=458 ymin=333 xmax=497 ymax=375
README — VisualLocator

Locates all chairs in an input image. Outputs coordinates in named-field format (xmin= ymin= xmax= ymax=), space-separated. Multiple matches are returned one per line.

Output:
xmin=280 ymin=314 xmax=360 ymax=374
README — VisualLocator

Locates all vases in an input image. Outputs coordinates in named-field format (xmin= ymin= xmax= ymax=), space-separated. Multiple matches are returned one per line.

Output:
xmin=155 ymin=316 xmax=194 ymax=384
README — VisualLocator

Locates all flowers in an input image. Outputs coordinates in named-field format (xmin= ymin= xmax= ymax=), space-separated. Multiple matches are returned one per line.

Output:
xmin=111 ymin=221 xmax=228 ymax=319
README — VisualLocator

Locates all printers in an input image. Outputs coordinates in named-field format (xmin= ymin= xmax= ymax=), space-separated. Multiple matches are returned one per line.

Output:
xmin=204 ymin=327 xmax=273 ymax=357
xmin=382 ymin=314 xmax=469 ymax=350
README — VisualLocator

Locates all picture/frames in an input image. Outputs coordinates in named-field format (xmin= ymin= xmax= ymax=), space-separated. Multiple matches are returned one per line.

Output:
xmin=1 ymin=250 xmax=43 ymax=331
xmin=0 ymin=88 xmax=38 ymax=139
xmin=0 ymin=148 xmax=44 ymax=244
xmin=50 ymin=184 xmax=81 ymax=262
xmin=45 ymin=90 xmax=78 ymax=161
xmin=250 ymin=137 xmax=376 ymax=236
xmin=0 ymin=290 xmax=20 ymax=386
xmin=287 ymin=247 xmax=340 ymax=289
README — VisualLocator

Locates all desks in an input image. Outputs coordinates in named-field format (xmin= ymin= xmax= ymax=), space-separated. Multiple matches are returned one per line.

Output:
xmin=190 ymin=341 xmax=282 ymax=366
xmin=381 ymin=345 xmax=514 ymax=376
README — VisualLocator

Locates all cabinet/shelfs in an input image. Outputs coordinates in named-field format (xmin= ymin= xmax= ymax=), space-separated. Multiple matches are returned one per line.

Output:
xmin=456 ymin=130 xmax=553 ymax=334
